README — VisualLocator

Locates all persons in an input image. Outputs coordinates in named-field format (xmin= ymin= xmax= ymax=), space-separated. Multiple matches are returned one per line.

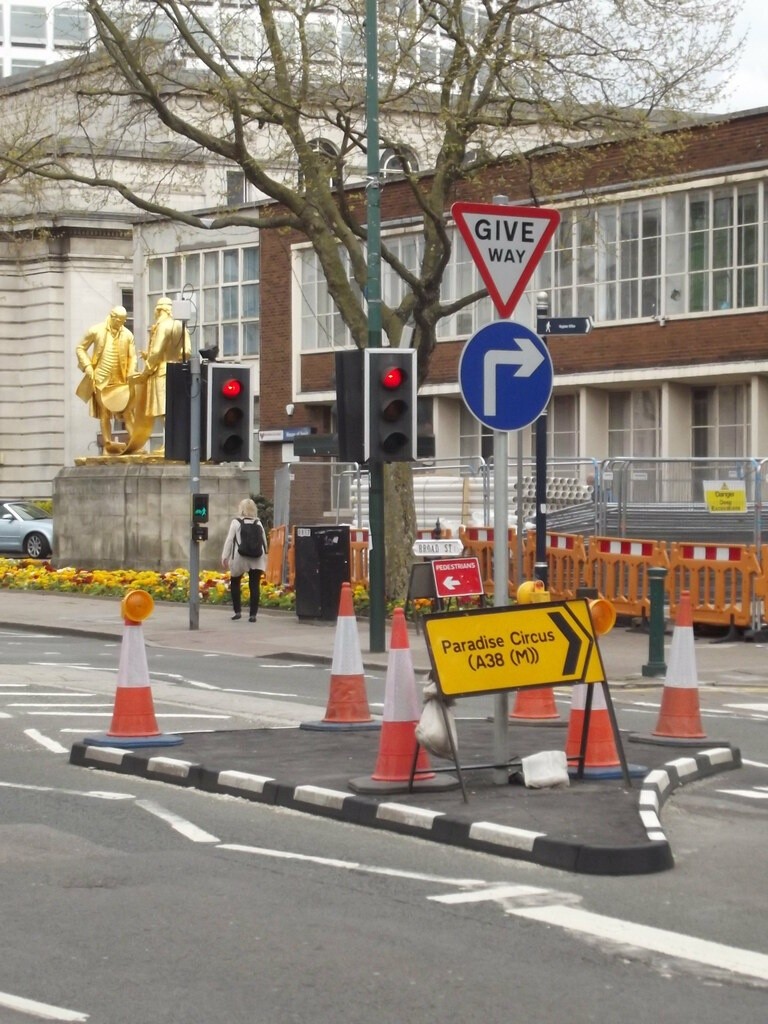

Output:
xmin=76 ymin=297 xmax=192 ymax=456
xmin=222 ymin=498 xmax=268 ymax=622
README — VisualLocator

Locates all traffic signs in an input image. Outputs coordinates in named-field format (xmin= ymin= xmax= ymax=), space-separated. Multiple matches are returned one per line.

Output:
xmin=430 ymin=557 xmax=484 ymax=597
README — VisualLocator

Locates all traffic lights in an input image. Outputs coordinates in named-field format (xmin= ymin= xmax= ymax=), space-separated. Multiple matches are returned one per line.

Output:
xmin=192 ymin=494 xmax=208 ymax=542
xmin=380 ymin=363 xmax=410 ymax=452
xmin=216 ymin=376 xmax=247 ymax=458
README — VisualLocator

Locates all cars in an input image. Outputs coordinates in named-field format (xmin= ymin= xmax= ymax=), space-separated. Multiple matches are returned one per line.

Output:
xmin=0 ymin=500 xmax=53 ymax=559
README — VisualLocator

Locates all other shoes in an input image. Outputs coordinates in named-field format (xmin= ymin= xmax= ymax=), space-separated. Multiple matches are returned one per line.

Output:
xmin=249 ymin=616 xmax=256 ymax=622
xmin=232 ymin=612 xmax=241 ymax=620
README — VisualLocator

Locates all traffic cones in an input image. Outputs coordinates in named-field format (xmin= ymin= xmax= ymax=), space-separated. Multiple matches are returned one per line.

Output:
xmin=627 ymin=590 xmax=731 ymax=748
xmin=83 ymin=587 xmax=185 ymax=747
xmin=298 ymin=581 xmax=383 ymax=731
xmin=347 ymin=607 xmax=462 ymax=795
xmin=485 ymin=687 xmax=568 ymax=728
xmin=565 ymin=684 xmax=648 ymax=781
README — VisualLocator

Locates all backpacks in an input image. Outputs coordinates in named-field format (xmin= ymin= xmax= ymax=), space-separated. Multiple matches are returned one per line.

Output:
xmin=232 ymin=517 xmax=268 ymax=559
